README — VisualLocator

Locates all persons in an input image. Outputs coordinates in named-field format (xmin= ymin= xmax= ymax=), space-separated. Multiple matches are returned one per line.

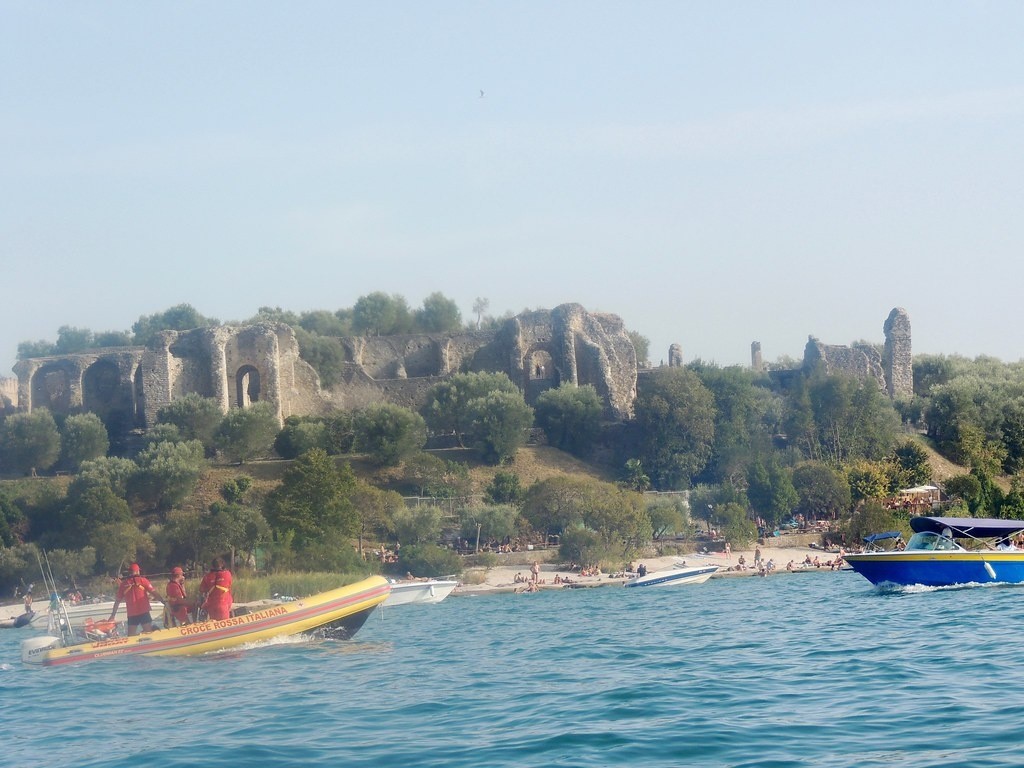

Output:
xmin=580 ymin=564 xmax=601 ymax=577
xmin=108 ymin=564 xmax=168 ymax=638
xmin=407 ymin=571 xmax=429 ymax=581
xmin=793 ymin=514 xmax=816 ymax=526
xmin=935 ymin=522 xmax=953 ymax=551
xmin=554 ymin=574 xmax=572 ymax=584
xmin=67 ymin=589 xmax=84 ymax=605
xmin=709 ymin=528 xmax=716 ymax=543
xmin=754 ymin=545 xmax=775 ymax=578
xmin=610 ymin=569 xmax=626 ymax=578
xmin=165 ymin=567 xmax=193 ymax=626
xmin=381 ymin=541 xmax=401 ymax=564
xmin=514 ymin=561 xmax=546 ymax=594
xmin=626 ymin=561 xmax=647 ymax=577
xmin=354 ymin=545 xmax=367 ymax=562
xmin=787 ymin=559 xmax=794 ymax=573
xmin=802 ymin=532 xmax=846 ymax=570
xmin=503 ymin=542 xmax=521 ymax=553
xmin=201 ymin=557 xmax=233 ymax=620
xmin=886 ymin=494 xmax=941 ymax=518
xmin=851 ymin=539 xmax=884 ymax=553
xmin=1013 ymin=532 xmax=1024 ymax=549
xmin=22 ymin=581 xmax=36 ymax=618
xmin=724 ymin=540 xmax=746 ymax=572
xmin=894 ymin=538 xmax=907 ymax=551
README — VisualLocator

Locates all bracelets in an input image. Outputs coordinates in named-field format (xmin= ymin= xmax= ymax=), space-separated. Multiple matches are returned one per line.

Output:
xmin=159 ymin=598 xmax=166 ymax=604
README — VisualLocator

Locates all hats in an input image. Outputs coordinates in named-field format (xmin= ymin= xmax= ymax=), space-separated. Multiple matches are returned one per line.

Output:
xmin=170 ymin=567 xmax=185 ymax=574
xmin=127 ymin=564 xmax=142 ymax=571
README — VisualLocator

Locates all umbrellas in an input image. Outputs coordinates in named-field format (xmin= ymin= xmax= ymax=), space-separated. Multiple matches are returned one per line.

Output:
xmin=917 ymin=484 xmax=939 ymax=494
xmin=900 ymin=486 xmax=929 ymax=498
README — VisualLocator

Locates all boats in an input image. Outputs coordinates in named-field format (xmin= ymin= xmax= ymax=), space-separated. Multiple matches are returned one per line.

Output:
xmin=0 ymin=599 xmax=165 ymax=633
xmin=840 ymin=516 xmax=1024 ymax=592
xmin=623 ymin=557 xmax=719 ymax=589
xmin=20 ymin=548 xmax=393 ymax=669
xmin=376 ymin=577 xmax=459 ymax=605
